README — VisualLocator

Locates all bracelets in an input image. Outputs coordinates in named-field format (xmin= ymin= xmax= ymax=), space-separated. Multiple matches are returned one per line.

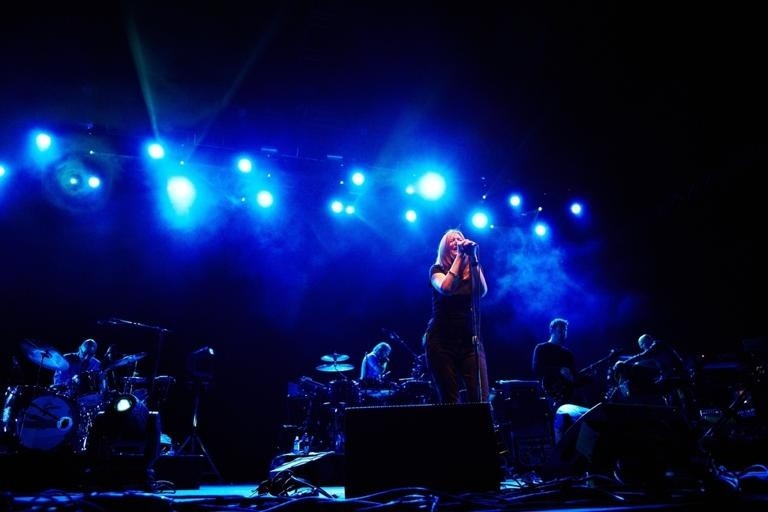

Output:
xmin=448 ymin=270 xmax=456 ymax=277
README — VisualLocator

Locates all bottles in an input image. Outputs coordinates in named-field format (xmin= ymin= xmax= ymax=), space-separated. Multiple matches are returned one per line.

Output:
xmin=294 ymin=435 xmax=299 ymax=452
xmin=303 ymin=432 xmax=310 ymax=455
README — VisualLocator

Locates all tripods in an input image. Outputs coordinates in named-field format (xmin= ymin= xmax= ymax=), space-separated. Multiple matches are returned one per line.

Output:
xmin=169 ymin=389 xmax=223 ymax=482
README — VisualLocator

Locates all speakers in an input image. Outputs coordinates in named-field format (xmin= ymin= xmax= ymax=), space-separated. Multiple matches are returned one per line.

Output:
xmin=559 ymin=401 xmax=672 ymax=479
xmin=343 ymin=402 xmax=498 ymax=498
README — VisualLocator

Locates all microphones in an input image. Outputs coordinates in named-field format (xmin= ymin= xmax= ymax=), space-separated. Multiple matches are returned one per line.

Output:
xmin=460 ymin=243 xmax=477 ymax=252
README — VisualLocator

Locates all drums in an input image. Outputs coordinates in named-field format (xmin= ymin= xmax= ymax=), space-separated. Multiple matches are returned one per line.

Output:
xmin=17 ymin=394 xmax=80 ymax=450
xmin=72 ymin=370 xmax=102 ymax=395
xmin=2 ymin=385 xmax=51 ymax=436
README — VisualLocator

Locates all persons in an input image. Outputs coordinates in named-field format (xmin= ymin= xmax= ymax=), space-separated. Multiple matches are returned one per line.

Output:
xmin=612 ymin=333 xmax=696 ymax=422
xmin=423 ymin=228 xmax=492 ymax=404
xmin=359 ymin=341 xmax=392 ymax=388
xmin=52 ymin=338 xmax=105 ymax=395
xmin=528 ymin=318 xmax=576 ymax=412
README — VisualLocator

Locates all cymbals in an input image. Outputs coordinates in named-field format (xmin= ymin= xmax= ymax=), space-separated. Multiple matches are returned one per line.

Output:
xmin=322 ymin=354 xmax=349 ymax=362
xmin=25 ymin=341 xmax=69 ymax=370
xmin=317 ymin=364 xmax=354 ymax=373
xmin=121 ymin=319 xmax=161 ymax=329
xmin=108 ymin=352 xmax=147 ymax=372
